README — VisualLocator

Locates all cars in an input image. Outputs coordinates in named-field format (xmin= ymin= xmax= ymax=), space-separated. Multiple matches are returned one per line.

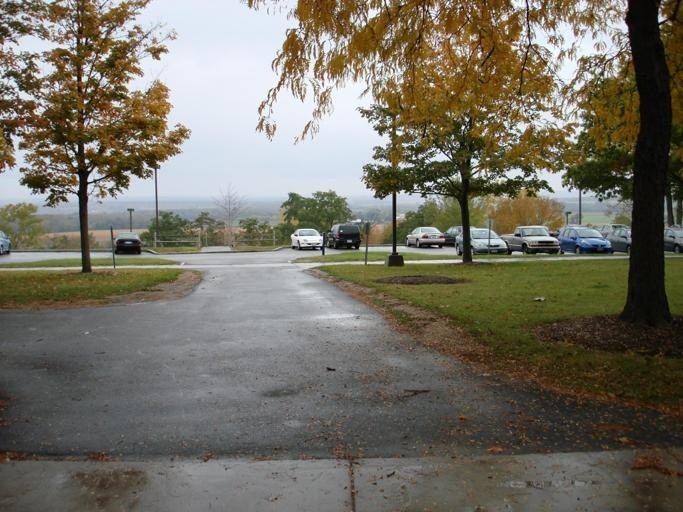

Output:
xmin=591 ymin=224 xmax=630 ymax=240
xmin=405 ymin=225 xmax=446 ymax=249
xmin=604 ymin=226 xmax=631 ymax=255
xmin=0 ymin=229 xmax=12 ymax=256
xmin=664 ymin=225 xmax=683 ymax=254
xmin=290 ymin=228 xmax=324 ymax=251
xmin=112 ymin=231 xmax=143 ymax=255
xmin=454 ymin=227 xmax=509 ymax=258
xmin=554 ymin=225 xmax=614 ymax=256
xmin=441 ymin=223 xmax=477 ymax=246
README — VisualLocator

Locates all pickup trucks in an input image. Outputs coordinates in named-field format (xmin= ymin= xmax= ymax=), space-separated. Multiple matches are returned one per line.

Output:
xmin=499 ymin=225 xmax=561 ymax=257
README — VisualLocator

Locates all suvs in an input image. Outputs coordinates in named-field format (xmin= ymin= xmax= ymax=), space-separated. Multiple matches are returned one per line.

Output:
xmin=326 ymin=223 xmax=362 ymax=250
